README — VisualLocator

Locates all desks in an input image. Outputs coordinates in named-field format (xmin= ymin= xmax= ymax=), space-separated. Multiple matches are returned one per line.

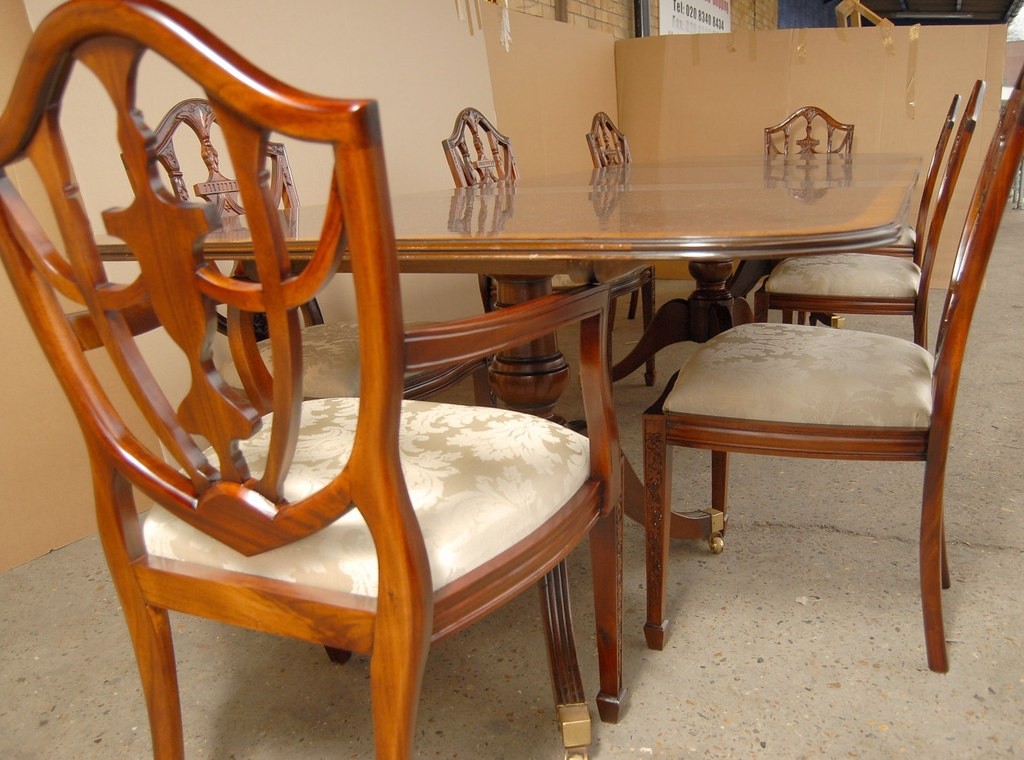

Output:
xmin=70 ymin=149 xmax=924 ymax=760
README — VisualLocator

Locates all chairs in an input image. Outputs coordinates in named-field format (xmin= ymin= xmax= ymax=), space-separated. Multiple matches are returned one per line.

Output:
xmin=765 ymin=106 xmax=854 ymax=156
xmin=755 ymin=79 xmax=986 ymax=350
xmin=119 ymin=97 xmax=504 ymax=665
xmin=642 ymin=62 xmax=1023 ymax=673
xmin=439 ymin=107 xmax=655 ymax=389
xmin=796 ymin=94 xmax=958 ymax=325
xmin=587 ymin=111 xmax=639 ymax=319
xmin=0 ymin=0 xmax=626 ymax=760
